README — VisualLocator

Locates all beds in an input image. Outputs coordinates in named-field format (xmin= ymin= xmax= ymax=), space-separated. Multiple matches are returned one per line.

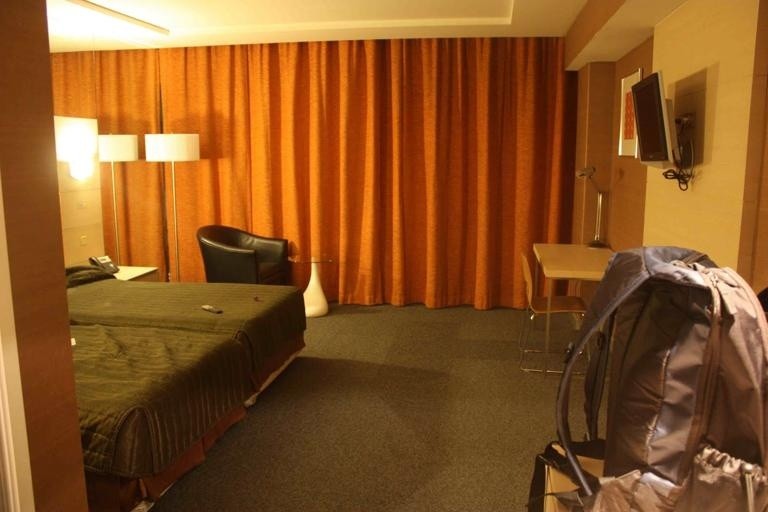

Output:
xmin=65 ymin=323 xmax=248 ymax=511
xmin=65 ymin=279 xmax=306 ymax=410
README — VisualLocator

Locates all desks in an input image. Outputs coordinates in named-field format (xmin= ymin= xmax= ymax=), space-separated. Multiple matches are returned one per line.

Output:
xmin=533 ymin=243 xmax=624 ymax=375
xmin=113 ymin=265 xmax=159 ymax=282
xmin=288 ymin=256 xmax=335 ymax=317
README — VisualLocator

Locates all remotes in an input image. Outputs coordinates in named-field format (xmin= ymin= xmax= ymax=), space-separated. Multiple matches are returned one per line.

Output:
xmin=202 ymin=304 xmax=222 ymax=313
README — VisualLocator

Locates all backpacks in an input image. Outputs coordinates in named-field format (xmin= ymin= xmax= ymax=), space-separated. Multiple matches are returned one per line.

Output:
xmin=557 ymin=245 xmax=766 ymax=510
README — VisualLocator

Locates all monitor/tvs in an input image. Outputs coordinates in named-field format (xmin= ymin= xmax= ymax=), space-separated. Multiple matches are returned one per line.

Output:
xmin=632 ymin=71 xmax=691 ymax=165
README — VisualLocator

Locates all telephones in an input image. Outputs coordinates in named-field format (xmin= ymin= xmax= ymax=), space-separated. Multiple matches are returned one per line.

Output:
xmin=88 ymin=255 xmax=119 ymax=274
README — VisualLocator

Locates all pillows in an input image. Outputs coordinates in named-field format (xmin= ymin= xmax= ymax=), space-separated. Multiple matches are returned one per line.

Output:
xmin=67 ymin=264 xmax=117 ymax=287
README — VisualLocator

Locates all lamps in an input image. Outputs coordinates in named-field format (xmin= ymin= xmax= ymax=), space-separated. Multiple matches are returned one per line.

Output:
xmin=144 ymin=133 xmax=200 ymax=282
xmin=98 ymin=135 xmax=139 ymax=265
xmin=576 ymin=166 xmax=613 ymax=248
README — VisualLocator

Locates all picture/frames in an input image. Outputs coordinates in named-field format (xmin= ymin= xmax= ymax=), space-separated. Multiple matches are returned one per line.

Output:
xmin=617 ymin=68 xmax=642 ymax=159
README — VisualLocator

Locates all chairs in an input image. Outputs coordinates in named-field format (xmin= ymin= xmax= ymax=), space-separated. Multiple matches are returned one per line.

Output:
xmin=196 ymin=224 xmax=288 ymax=284
xmin=519 ymin=255 xmax=591 ymax=376
